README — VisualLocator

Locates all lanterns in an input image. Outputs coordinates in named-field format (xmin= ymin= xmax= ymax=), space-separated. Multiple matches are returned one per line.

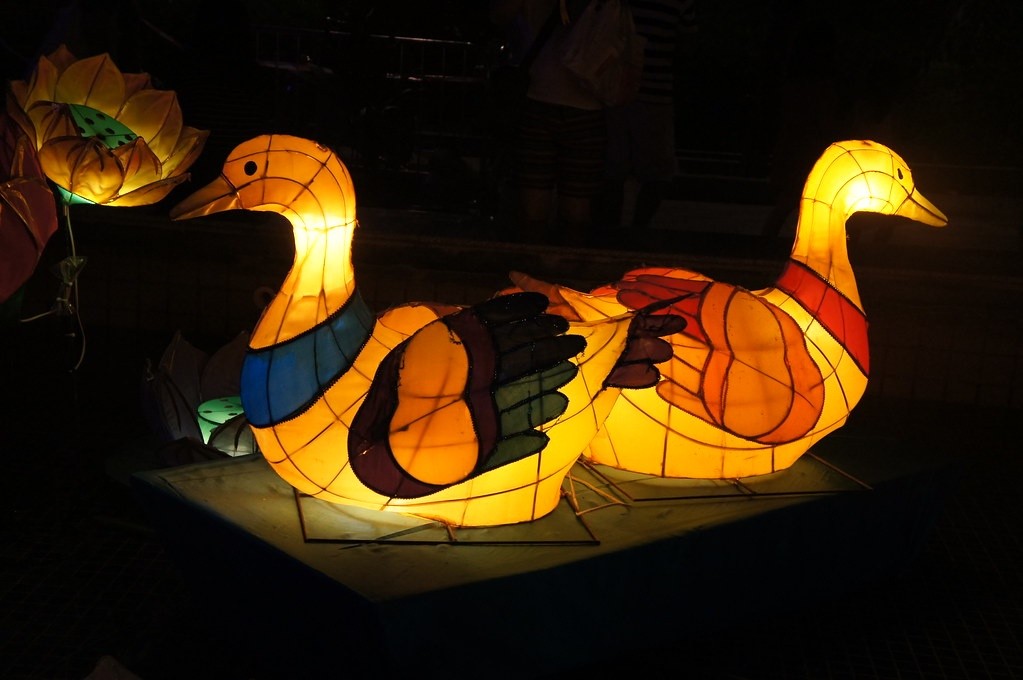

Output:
xmin=169 ymin=132 xmax=951 ymax=524
xmin=0 ymin=43 xmax=211 ymax=303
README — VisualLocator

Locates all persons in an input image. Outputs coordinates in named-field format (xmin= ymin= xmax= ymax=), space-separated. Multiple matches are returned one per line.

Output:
xmin=488 ymin=0 xmax=708 ymax=233
